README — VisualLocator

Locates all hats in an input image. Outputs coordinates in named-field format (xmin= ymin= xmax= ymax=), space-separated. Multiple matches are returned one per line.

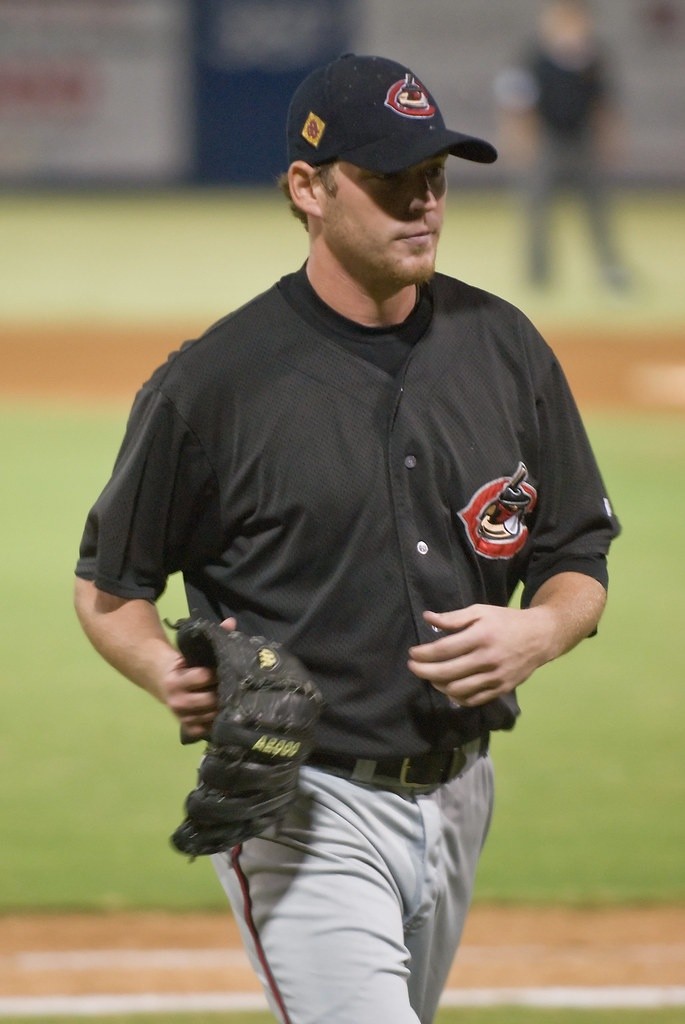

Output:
xmin=286 ymin=53 xmax=498 ymax=178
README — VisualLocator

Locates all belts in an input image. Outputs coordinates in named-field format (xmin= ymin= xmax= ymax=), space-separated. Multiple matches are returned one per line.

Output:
xmin=301 ymin=732 xmax=490 ymax=793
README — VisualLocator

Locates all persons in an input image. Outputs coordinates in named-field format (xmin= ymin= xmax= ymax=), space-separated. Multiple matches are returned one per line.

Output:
xmin=501 ymin=0 xmax=636 ymax=306
xmin=73 ymin=51 xmax=622 ymax=1021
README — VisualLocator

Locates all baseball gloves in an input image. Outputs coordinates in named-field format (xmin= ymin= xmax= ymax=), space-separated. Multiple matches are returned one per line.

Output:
xmin=171 ymin=614 xmax=323 ymax=855
